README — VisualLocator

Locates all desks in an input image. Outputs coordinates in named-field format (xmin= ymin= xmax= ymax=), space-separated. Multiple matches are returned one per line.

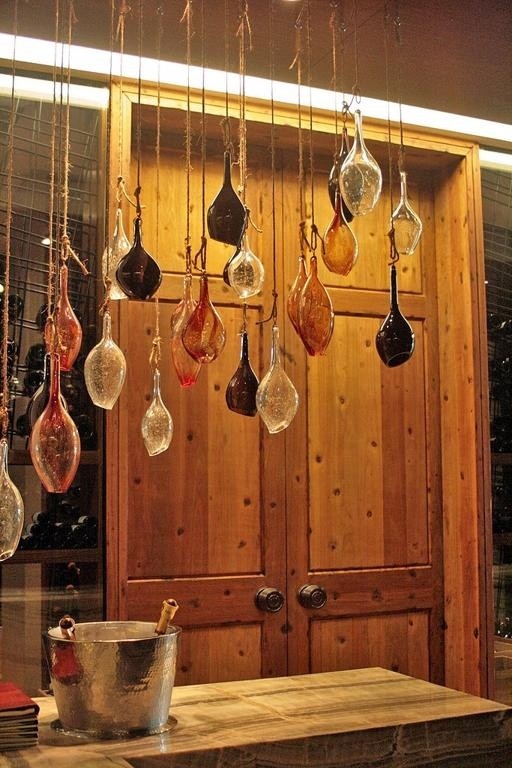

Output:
xmin=0 ymin=666 xmax=510 ymax=768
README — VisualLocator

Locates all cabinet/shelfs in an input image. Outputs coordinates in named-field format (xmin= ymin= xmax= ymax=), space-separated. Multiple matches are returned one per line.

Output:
xmin=108 ymin=79 xmax=496 ymax=701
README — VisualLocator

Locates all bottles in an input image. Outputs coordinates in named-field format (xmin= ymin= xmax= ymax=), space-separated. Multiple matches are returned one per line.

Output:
xmin=59 ymin=616 xmax=77 ymax=642
xmin=16 ymin=485 xmax=96 ymax=550
xmin=154 ymin=598 xmax=179 ymax=638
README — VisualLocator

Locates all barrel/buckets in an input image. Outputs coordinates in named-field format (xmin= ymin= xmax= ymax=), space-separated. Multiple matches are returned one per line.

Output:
xmin=45 ymin=619 xmax=183 ymax=733
